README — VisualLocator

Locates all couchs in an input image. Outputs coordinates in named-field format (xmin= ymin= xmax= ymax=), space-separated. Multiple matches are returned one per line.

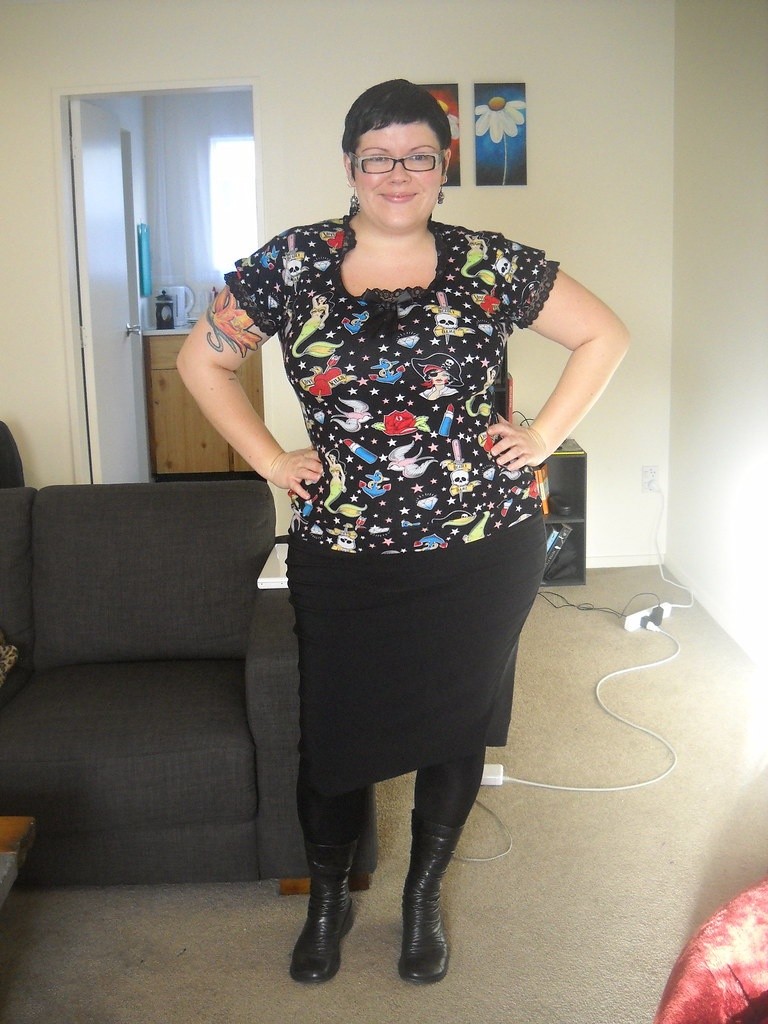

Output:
xmin=0 ymin=483 xmax=378 ymax=897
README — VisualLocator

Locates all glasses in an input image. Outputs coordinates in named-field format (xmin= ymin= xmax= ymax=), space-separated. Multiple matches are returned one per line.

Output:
xmin=345 ymin=149 xmax=443 ymax=175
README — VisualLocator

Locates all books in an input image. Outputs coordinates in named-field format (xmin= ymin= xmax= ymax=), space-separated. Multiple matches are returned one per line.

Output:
xmin=543 ymin=523 xmax=572 ymax=582
xmin=533 ymin=464 xmax=551 ymax=515
xmin=554 ymin=439 xmax=585 ymax=455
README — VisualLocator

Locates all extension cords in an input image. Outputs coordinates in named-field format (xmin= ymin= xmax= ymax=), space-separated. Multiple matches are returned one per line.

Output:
xmin=624 ymin=602 xmax=672 ymax=632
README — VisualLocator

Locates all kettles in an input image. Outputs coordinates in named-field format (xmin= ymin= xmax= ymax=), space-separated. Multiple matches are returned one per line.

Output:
xmin=156 ymin=286 xmax=194 ymax=326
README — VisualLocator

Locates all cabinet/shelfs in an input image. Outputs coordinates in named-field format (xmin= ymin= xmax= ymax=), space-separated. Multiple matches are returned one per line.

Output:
xmin=539 ymin=439 xmax=588 ymax=587
xmin=143 ymin=328 xmax=265 ymax=481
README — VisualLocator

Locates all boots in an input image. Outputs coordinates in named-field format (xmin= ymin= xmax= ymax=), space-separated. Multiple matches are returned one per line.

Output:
xmin=290 ymin=839 xmax=358 ymax=984
xmin=398 ymin=808 xmax=465 ymax=983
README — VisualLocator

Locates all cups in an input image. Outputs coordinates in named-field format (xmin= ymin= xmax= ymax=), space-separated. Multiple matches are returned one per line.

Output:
xmin=153 ymin=290 xmax=173 ymax=330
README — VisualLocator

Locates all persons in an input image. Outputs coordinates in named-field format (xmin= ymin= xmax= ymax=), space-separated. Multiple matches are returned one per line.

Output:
xmin=174 ymin=80 xmax=630 ymax=985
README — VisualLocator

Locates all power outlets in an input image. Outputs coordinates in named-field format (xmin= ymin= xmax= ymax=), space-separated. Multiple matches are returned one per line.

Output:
xmin=643 ymin=465 xmax=659 ymax=493
